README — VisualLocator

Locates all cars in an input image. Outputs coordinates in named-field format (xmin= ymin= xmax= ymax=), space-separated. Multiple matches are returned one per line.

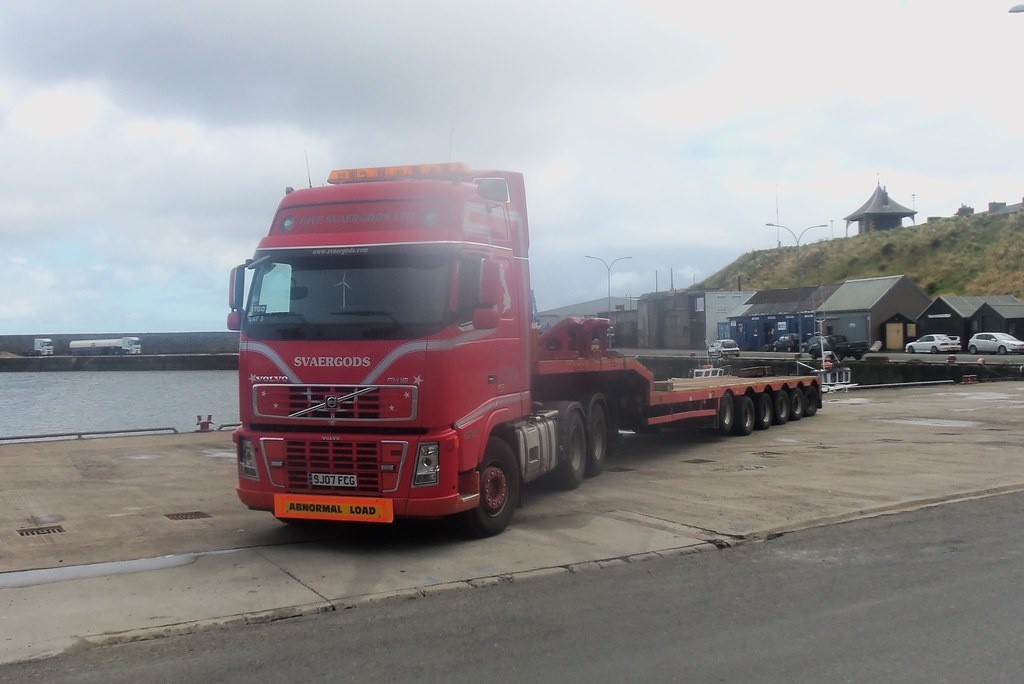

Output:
xmin=967 ymin=332 xmax=1024 ymax=356
xmin=905 ymin=334 xmax=962 ymax=354
xmin=708 ymin=339 xmax=741 ymax=358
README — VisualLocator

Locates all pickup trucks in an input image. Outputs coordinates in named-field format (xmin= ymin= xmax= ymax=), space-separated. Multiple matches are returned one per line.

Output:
xmin=800 ymin=335 xmax=870 ymax=360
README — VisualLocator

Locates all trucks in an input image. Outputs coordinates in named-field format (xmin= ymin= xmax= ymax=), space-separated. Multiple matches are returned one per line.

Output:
xmin=17 ymin=338 xmax=54 ymax=356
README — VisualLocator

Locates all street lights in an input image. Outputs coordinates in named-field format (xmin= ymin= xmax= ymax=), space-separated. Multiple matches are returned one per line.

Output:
xmin=586 ymin=255 xmax=633 ymax=351
xmin=766 ymin=223 xmax=828 ymax=359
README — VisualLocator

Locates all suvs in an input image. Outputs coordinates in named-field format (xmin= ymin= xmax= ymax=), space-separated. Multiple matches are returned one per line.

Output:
xmin=771 ymin=332 xmax=808 ymax=353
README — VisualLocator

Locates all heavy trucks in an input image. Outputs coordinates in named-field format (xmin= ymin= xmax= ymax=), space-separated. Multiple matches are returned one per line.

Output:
xmin=226 ymin=163 xmax=823 ymax=539
xmin=68 ymin=336 xmax=142 ymax=357
